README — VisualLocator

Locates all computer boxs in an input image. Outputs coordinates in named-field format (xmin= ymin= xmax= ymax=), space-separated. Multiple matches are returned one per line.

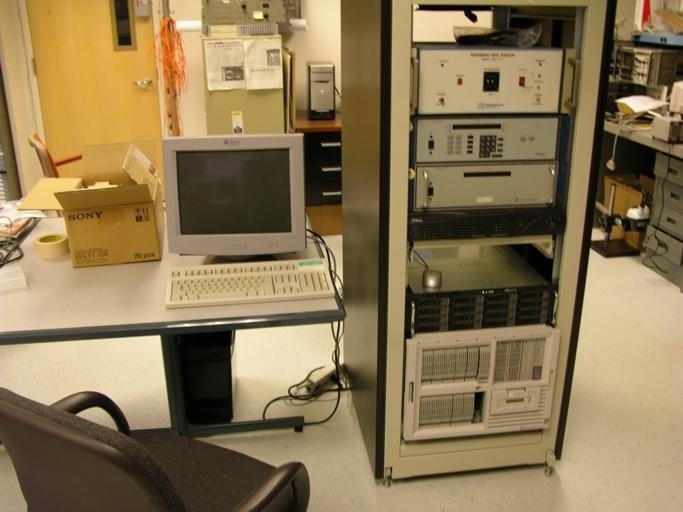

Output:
xmin=307 ymin=61 xmax=336 ymax=120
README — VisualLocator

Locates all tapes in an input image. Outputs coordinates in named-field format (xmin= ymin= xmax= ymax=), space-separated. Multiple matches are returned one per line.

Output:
xmin=36 ymin=233 xmax=68 ymax=261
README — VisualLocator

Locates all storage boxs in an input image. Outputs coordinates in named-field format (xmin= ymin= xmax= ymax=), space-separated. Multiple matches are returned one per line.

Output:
xmin=16 ymin=139 xmax=165 ymax=269
xmin=601 ymin=174 xmax=654 ymax=250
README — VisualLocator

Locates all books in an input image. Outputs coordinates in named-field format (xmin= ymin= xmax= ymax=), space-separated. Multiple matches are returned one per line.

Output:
xmin=1 ymin=209 xmax=47 ymax=239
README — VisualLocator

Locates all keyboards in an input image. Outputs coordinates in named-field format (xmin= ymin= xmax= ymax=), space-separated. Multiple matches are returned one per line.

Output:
xmin=165 ymin=258 xmax=335 ymax=310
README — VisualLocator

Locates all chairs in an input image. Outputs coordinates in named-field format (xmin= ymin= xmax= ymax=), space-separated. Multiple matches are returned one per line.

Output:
xmin=27 ymin=132 xmax=82 ymax=177
xmin=0 ymin=387 xmax=309 ymax=512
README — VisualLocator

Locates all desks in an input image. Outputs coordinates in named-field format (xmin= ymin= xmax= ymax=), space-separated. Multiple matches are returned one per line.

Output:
xmin=0 ymin=209 xmax=347 ymax=432
xmin=592 ymin=122 xmax=682 ymax=292
xmin=295 ymin=110 xmax=341 ymax=206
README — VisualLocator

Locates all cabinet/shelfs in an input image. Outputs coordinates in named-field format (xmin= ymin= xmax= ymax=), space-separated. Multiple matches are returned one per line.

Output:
xmin=340 ymin=0 xmax=618 ymax=483
xmin=199 ymin=34 xmax=285 ymax=137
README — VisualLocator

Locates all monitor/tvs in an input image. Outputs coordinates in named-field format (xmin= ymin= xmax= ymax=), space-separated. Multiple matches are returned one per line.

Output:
xmin=160 ymin=133 xmax=304 ymax=264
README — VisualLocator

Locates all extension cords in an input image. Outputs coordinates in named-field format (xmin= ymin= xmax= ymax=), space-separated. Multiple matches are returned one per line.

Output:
xmin=304 ymin=358 xmax=345 ymax=394
